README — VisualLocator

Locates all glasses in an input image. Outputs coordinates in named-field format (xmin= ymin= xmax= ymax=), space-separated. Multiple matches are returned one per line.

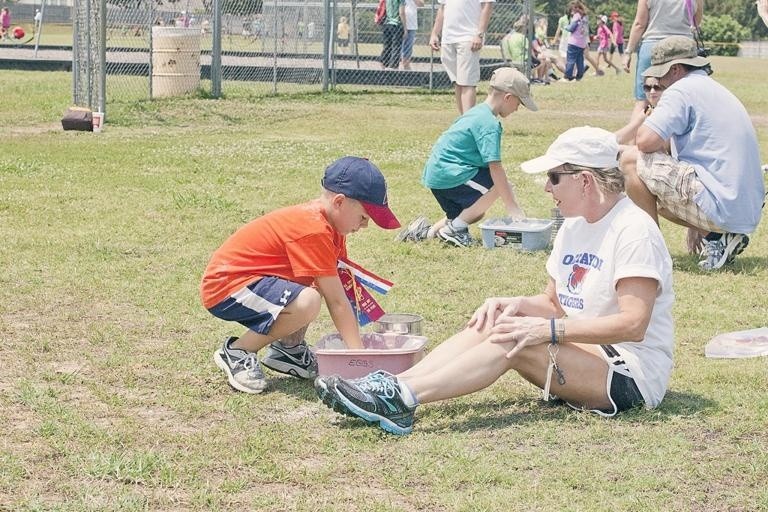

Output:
xmin=643 ymin=85 xmax=660 ymax=92
xmin=547 ymin=171 xmax=577 ymax=186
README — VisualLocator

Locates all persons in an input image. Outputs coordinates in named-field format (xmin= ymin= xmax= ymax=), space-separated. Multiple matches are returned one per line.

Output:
xmin=618 ymin=35 xmax=765 ymax=269
xmin=499 ymin=1 xmax=624 ymax=84
xmin=401 ymin=1 xmax=423 ymax=70
xmin=756 ymin=0 xmax=767 ymax=29
xmin=199 ymin=155 xmax=401 ymax=395
xmin=429 ymin=1 xmax=492 ymax=115
xmin=0 ymin=6 xmax=10 ymax=40
xmin=395 ymin=65 xmax=538 ymax=251
xmin=378 ymin=1 xmax=408 ymax=70
xmin=612 ymin=76 xmax=668 ymax=157
xmin=623 ymin=0 xmax=704 ymax=118
xmin=106 ymin=9 xmax=350 ymax=56
xmin=34 ymin=8 xmax=41 ymax=28
xmin=312 ymin=126 xmax=674 ymax=435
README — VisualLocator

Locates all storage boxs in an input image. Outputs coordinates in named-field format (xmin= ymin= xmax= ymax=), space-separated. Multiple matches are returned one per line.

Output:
xmin=478 ymin=217 xmax=555 ymax=251
xmin=311 ymin=333 xmax=426 ymax=380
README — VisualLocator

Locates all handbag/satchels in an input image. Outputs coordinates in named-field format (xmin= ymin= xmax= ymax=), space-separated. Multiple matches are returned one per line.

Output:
xmin=375 ymin=0 xmax=387 ymax=29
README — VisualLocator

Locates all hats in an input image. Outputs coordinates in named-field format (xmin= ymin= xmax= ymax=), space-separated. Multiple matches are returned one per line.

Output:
xmin=521 ymin=126 xmax=621 ymax=173
xmin=641 ymin=35 xmax=710 ymax=77
xmin=489 ymin=67 xmax=537 ymax=111
xmin=322 ymin=156 xmax=401 ymax=228
xmin=596 ymin=15 xmax=607 ymax=23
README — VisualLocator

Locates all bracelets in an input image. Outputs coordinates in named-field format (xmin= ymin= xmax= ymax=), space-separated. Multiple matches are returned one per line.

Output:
xmin=550 ymin=317 xmax=555 ymax=344
xmin=625 ymin=48 xmax=632 ymax=54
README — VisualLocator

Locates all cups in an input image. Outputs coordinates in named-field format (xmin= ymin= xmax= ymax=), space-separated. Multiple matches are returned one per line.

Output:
xmin=92 ymin=112 xmax=104 ymax=132
xmin=550 ymin=207 xmax=565 ymax=241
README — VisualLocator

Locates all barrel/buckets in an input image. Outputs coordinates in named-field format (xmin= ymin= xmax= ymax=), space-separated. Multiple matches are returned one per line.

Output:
xmin=151 ymin=25 xmax=203 ymax=99
xmin=550 ymin=206 xmax=565 ymax=248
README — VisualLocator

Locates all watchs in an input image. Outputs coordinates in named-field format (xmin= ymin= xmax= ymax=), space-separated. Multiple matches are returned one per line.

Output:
xmin=478 ymin=34 xmax=484 ymax=38
xmin=557 ymin=322 xmax=564 ymax=344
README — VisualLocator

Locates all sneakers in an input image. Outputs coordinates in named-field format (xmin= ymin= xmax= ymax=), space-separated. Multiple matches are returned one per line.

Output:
xmin=698 ymin=232 xmax=749 ymax=271
xmin=393 ymin=216 xmax=432 ymax=243
xmin=591 ymin=63 xmax=631 ymax=76
xmin=437 ymin=221 xmax=484 ymax=249
xmin=213 ymin=336 xmax=267 ymax=393
xmin=260 ymin=340 xmax=317 ymax=379
xmin=314 ymin=369 xmax=419 ymax=436
xmin=531 ymin=66 xmax=588 ymax=85
